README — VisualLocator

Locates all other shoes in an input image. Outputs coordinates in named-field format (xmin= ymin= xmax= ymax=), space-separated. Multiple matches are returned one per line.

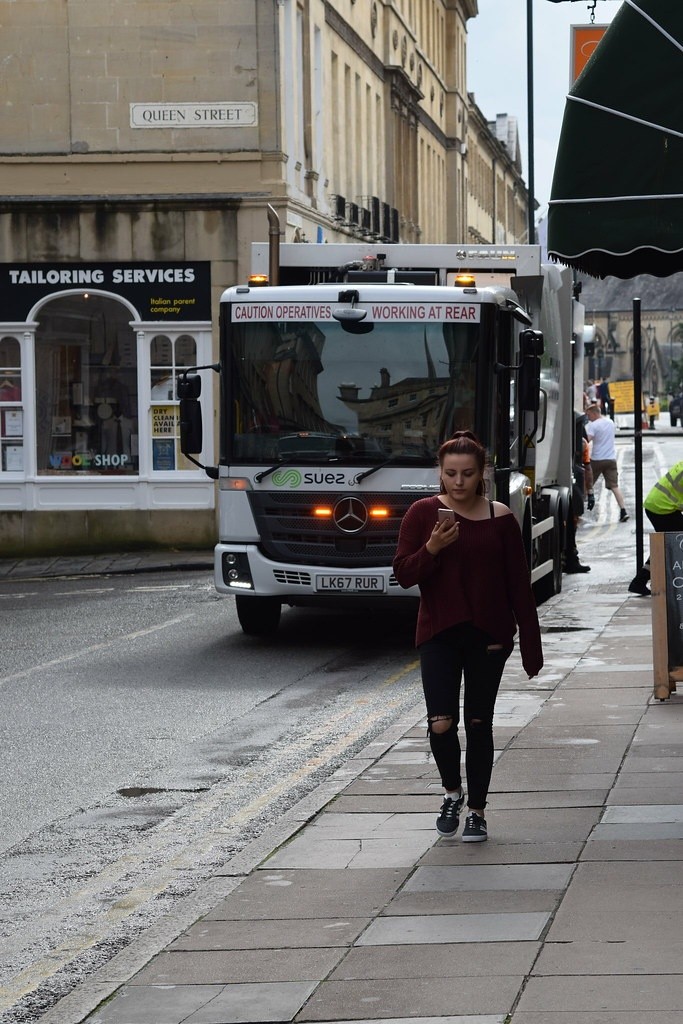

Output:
xmin=619 ymin=512 xmax=629 ymax=522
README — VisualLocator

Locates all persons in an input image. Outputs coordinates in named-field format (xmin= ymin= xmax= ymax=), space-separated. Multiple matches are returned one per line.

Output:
xmin=393 ymin=430 xmax=544 ymax=842
xmin=561 ymin=377 xmax=683 ymax=596
xmin=90 ymin=367 xmax=131 ymax=460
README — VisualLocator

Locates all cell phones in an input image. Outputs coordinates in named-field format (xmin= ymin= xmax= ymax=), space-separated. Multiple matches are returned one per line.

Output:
xmin=438 ymin=508 xmax=455 ymax=533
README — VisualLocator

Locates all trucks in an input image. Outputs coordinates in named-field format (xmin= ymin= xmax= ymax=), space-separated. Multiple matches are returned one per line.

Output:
xmin=173 ymin=236 xmax=600 ymax=634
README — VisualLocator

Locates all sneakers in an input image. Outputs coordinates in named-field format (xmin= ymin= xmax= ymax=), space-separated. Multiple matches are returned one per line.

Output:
xmin=435 ymin=786 xmax=465 ymax=837
xmin=462 ymin=812 xmax=488 ymax=842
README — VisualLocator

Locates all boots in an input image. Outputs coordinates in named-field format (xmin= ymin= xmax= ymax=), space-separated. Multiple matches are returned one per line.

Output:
xmin=628 ymin=567 xmax=651 ymax=595
xmin=561 ymin=549 xmax=569 ymax=573
xmin=564 ymin=546 xmax=590 ymax=574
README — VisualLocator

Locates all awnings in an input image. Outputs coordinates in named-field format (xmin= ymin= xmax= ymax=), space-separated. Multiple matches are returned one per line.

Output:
xmin=547 ymin=0 xmax=683 ymax=281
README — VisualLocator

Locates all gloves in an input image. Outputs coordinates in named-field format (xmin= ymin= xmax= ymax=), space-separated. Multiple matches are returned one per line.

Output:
xmin=587 ymin=493 xmax=594 ymax=511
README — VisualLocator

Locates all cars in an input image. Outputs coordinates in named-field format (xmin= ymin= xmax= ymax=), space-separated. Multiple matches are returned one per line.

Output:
xmin=669 ymin=379 xmax=683 ymax=428
